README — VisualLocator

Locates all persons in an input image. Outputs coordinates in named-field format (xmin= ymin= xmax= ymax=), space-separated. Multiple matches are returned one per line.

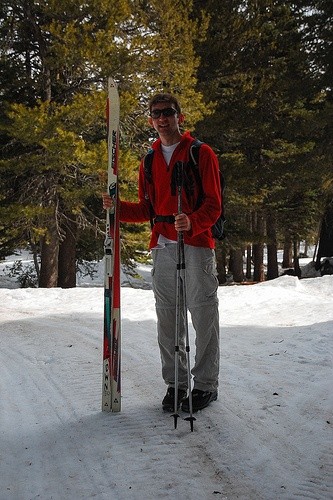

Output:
xmin=102 ymin=93 xmax=221 ymax=415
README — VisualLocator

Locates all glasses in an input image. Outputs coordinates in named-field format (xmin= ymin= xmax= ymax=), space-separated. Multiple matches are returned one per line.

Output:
xmin=149 ymin=106 xmax=178 ymax=119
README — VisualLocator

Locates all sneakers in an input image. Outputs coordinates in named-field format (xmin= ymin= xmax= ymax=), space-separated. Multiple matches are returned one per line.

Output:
xmin=161 ymin=387 xmax=187 ymax=408
xmin=182 ymin=388 xmax=218 ymax=413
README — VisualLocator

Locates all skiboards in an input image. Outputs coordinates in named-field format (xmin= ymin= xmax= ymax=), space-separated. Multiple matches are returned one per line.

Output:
xmin=101 ymin=75 xmax=122 ymax=412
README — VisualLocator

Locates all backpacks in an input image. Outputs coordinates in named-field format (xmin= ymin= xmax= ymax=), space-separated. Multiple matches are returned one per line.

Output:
xmin=144 ymin=138 xmax=225 ymax=240
